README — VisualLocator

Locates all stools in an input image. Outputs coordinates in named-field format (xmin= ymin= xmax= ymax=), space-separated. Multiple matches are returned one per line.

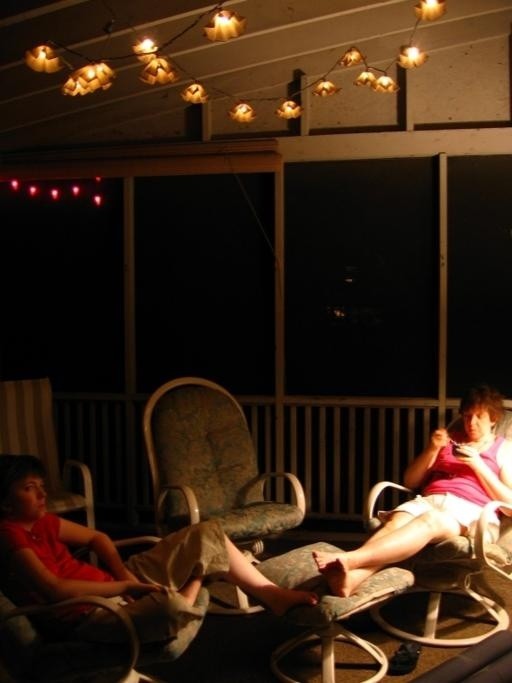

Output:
xmin=255 ymin=541 xmax=415 ymax=683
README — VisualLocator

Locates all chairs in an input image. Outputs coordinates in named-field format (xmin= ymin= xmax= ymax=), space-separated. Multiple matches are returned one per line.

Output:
xmin=363 ymin=399 xmax=512 ymax=648
xmin=0 ymin=536 xmax=209 ymax=683
xmin=142 ymin=377 xmax=306 ymax=616
xmin=0 ymin=378 xmax=97 ymax=566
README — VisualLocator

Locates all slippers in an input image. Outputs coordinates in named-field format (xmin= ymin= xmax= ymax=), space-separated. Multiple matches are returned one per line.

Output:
xmin=387 ymin=641 xmax=422 ymax=676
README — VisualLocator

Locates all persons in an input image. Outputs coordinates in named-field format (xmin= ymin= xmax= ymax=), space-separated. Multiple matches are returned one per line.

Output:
xmin=311 ymin=382 xmax=512 ymax=598
xmin=0 ymin=455 xmax=321 ymax=646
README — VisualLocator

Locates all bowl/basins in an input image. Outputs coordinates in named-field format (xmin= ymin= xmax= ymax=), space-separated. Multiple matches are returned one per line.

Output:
xmin=452 ymin=444 xmax=470 ymax=458
